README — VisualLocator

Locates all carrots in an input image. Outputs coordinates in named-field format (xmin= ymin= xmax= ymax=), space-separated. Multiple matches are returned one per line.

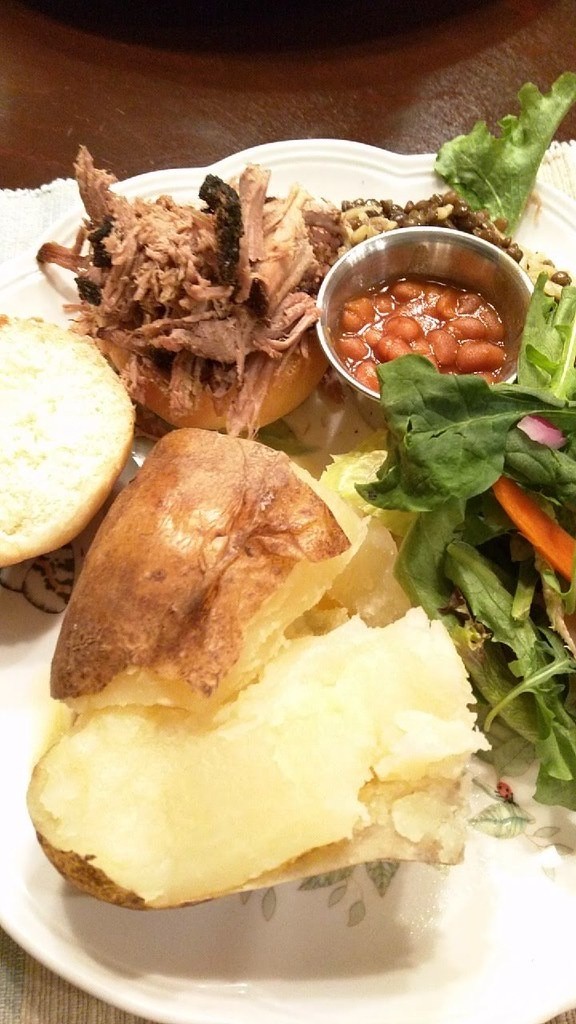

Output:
xmin=493 ymin=475 xmax=576 ymax=584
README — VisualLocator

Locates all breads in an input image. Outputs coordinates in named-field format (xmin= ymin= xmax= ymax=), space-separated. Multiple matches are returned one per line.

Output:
xmin=0 ymin=312 xmax=134 ymax=565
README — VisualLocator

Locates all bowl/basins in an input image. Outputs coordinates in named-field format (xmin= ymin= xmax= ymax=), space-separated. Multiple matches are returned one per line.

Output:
xmin=315 ymin=224 xmax=535 ymax=403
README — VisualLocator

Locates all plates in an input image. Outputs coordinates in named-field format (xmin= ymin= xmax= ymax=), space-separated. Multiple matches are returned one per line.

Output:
xmin=0 ymin=137 xmax=576 ymax=1024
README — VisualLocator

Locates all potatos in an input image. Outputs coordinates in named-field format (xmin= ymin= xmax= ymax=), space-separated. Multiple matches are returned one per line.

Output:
xmin=25 ymin=427 xmax=493 ymax=908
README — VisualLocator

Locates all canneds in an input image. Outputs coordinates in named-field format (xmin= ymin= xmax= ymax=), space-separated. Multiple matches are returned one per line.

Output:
xmin=102 ymin=327 xmax=329 ymax=434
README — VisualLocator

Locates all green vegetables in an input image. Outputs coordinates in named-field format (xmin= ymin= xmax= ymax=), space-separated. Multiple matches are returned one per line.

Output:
xmin=355 ymin=72 xmax=576 ymax=818
